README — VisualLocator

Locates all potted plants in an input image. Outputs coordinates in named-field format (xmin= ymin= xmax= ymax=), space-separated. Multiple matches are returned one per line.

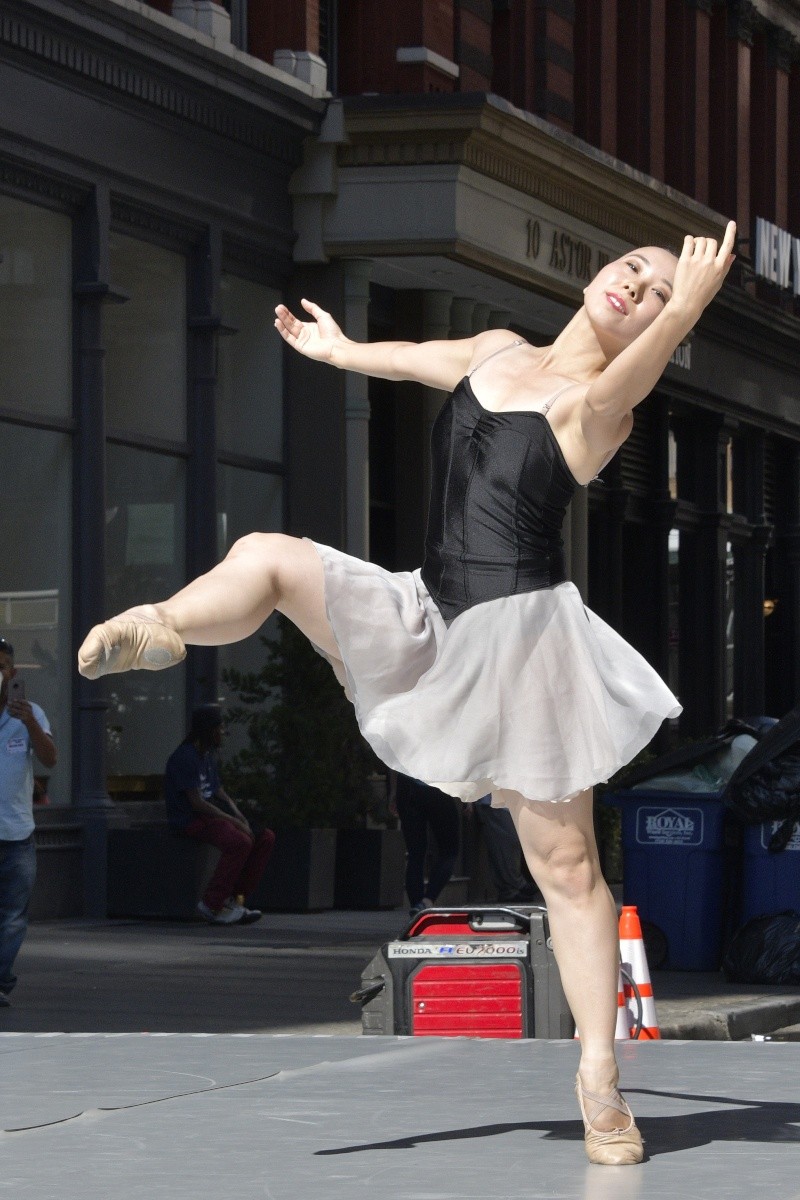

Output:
xmin=230 ymin=616 xmax=379 ymax=909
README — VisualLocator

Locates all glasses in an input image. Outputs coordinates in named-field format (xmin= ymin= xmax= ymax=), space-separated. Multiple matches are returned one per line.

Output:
xmin=1 ymin=638 xmax=9 ymax=648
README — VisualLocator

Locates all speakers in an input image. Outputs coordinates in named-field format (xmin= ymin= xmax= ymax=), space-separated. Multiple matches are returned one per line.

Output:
xmin=359 ymin=902 xmax=576 ymax=1040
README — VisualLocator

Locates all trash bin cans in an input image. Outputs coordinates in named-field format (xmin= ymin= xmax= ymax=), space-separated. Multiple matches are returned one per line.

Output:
xmin=722 ymin=709 xmax=800 ymax=918
xmin=601 ymin=734 xmax=736 ymax=973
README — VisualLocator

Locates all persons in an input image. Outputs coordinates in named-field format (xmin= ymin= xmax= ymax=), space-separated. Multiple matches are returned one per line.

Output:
xmin=77 ymin=215 xmax=736 ymax=1164
xmin=0 ymin=640 xmax=62 ymax=1007
xmin=386 ymin=767 xmax=473 ymax=918
xmin=164 ymin=703 xmax=275 ymax=925
xmin=474 ymin=794 xmax=539 ymax=903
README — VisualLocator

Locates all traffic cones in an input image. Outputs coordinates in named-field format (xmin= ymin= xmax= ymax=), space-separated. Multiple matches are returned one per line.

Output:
xmin=572 ymin=961 xmax=632 ymax=1040
xmin=617 ymin=906 xmax=661 ymax=1039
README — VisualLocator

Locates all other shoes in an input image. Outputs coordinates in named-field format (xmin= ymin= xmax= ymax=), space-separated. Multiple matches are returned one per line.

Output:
xmin=498 ymin=883 xmax=538 ymax=902
xmin=0 ymin=992 xmax=11 ymax=1005
xmin=409 ymin=902 xmax=425 ymax=919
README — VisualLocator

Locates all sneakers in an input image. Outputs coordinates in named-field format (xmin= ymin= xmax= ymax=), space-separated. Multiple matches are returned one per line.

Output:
xmin=197 ymin=900 xmax=233 ymax=923
xmin=224 ymin=903 xmax=262 ymax=925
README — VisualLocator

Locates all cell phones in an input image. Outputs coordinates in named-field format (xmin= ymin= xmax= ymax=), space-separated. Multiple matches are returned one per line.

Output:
xmin=8 ymin=679 xmax=25 ymax=704
